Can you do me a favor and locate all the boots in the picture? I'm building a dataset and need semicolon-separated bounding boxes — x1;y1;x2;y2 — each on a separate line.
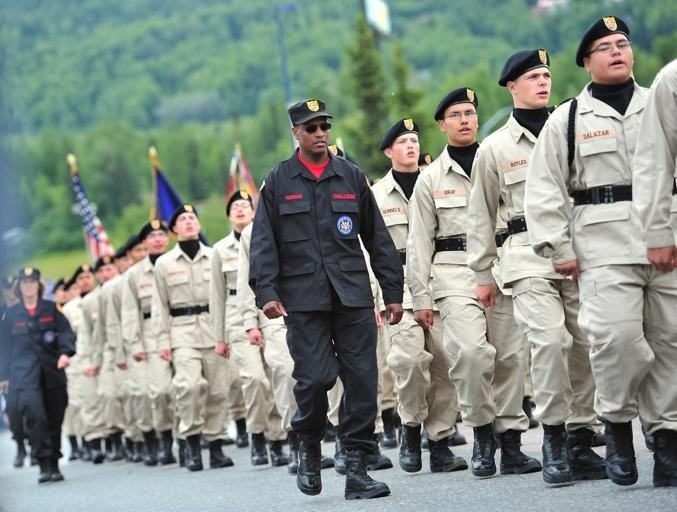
380;410;398;449
603;420;639;486
427;438;468;472
565;424;610;480
288;431;299;474
269;440;289;467
208;439;234;469
470;422;498;479
71;429;186;468
500;429;542;475
14;436;64;483
367;448;393;471
186;433;204;471
234;418;249;448
251;431;269;465
320;454;334;469
334;431;345;475
651;429;675;488
345;449;391;499
297;440;322;496
540;423;574;487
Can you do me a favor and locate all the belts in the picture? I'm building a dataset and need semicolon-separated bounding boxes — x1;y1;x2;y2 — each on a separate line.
170;305;208;317
143;312;151;319
229;289;236;295
395;185;633;266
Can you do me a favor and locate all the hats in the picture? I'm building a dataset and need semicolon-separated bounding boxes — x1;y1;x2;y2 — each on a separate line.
287;98;332;127
378;16;631;151
226;190;254;216
1;203;198;295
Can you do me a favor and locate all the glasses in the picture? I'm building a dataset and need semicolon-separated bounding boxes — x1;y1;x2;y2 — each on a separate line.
297;121;332;132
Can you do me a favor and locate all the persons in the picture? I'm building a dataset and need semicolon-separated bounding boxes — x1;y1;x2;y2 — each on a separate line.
50;220;187;467
468;48;607;480
407;87;543;478
208;191;289;467
629;58;677;271
521;15;677;489
2;267;76;480
150;204;234;471
360;117;469;475
249;99;406;500
237;221;335;475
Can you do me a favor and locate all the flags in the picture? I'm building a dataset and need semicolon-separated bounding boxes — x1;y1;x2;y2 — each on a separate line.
225;149;261;221
150;158;210;247
70;165;116;271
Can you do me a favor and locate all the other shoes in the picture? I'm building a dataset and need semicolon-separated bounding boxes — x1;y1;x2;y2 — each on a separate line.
324;424;336;442
591;430;605;447
447;424;468;446
523;408;540;429
